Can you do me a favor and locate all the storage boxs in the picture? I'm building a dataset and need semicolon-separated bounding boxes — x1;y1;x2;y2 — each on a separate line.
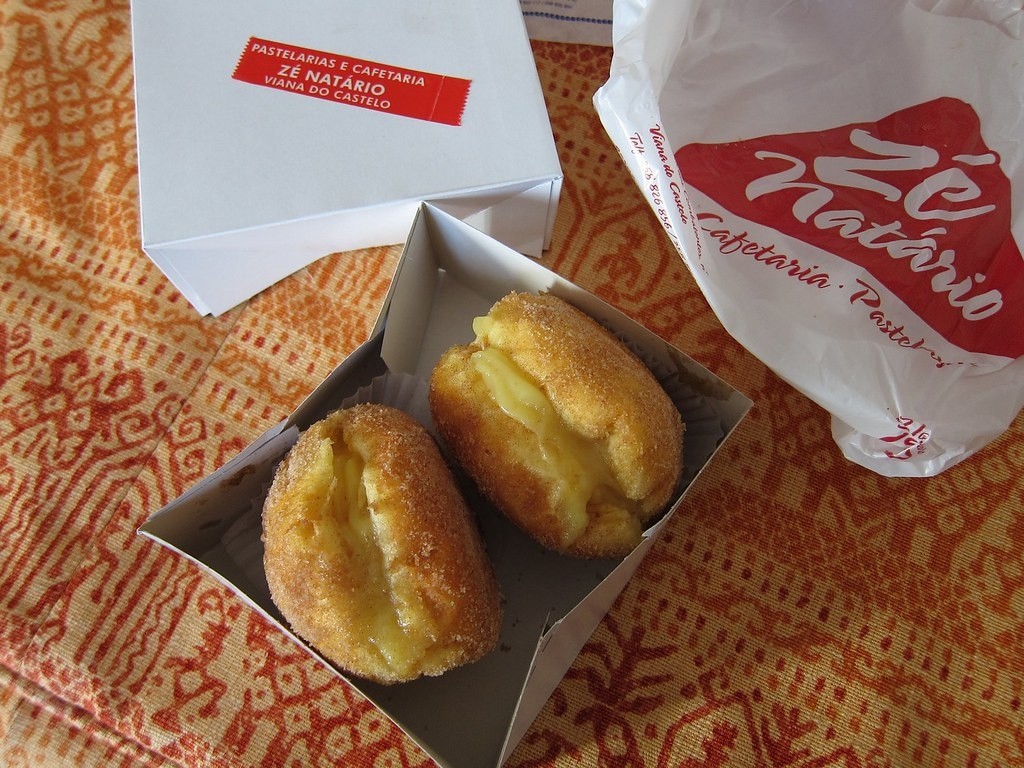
135;200;756;768
130;0;563;319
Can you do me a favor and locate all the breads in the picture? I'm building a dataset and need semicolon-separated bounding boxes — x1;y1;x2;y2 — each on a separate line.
431;294;686;562
259;402;502;685
